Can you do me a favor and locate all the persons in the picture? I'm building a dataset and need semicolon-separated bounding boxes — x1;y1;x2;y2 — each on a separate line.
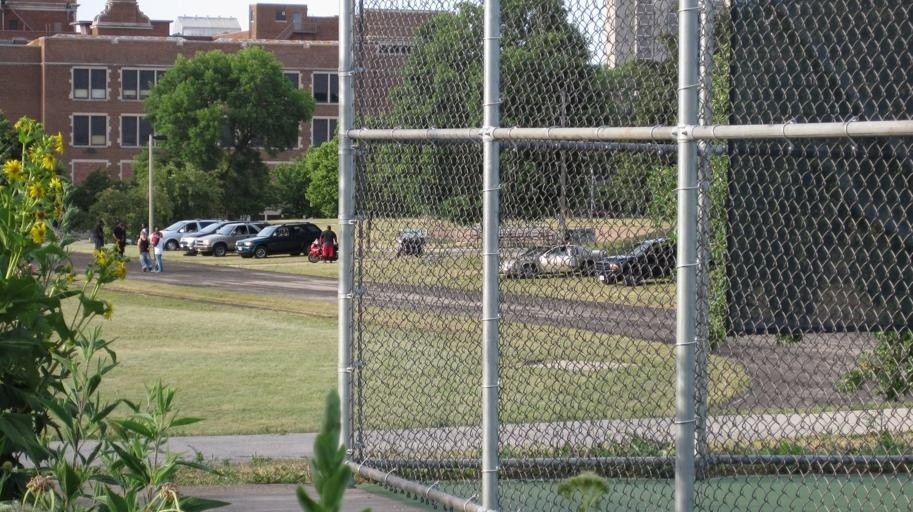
150;224;162;262
93;221;106;253
112;223;126;257
319;226;337;263
139;224;148;236
135;232;150;273
150;231;164;272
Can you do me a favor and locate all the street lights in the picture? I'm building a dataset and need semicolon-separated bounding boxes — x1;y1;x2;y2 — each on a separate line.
148;133;168;239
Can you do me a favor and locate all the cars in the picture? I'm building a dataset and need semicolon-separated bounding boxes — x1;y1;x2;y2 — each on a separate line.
499;239;679;285
163;219;323;260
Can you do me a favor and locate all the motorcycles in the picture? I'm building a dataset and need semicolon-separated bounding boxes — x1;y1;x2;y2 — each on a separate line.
308;239;338;263
